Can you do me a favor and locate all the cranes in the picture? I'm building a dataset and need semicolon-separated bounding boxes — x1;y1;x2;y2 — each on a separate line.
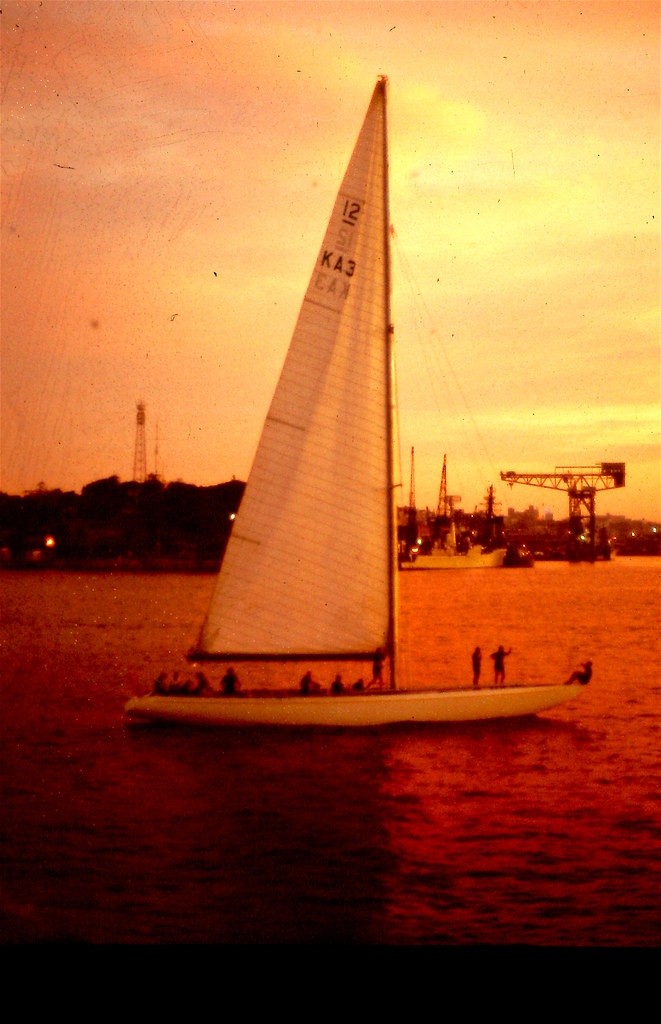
501;462;626;549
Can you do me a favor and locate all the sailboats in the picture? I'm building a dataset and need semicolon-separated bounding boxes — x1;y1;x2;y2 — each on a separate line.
125;72;585;727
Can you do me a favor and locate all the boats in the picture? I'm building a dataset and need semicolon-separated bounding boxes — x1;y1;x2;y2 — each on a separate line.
399;520;509;569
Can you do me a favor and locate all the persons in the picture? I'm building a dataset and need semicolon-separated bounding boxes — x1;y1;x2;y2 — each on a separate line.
564;661;593;686
351;681;363;690
489;644;512;687
471;645;483;686
300;671;312;692
220;667;237;696
331;675;345;695
366;646;386;689
153;669;208;697
